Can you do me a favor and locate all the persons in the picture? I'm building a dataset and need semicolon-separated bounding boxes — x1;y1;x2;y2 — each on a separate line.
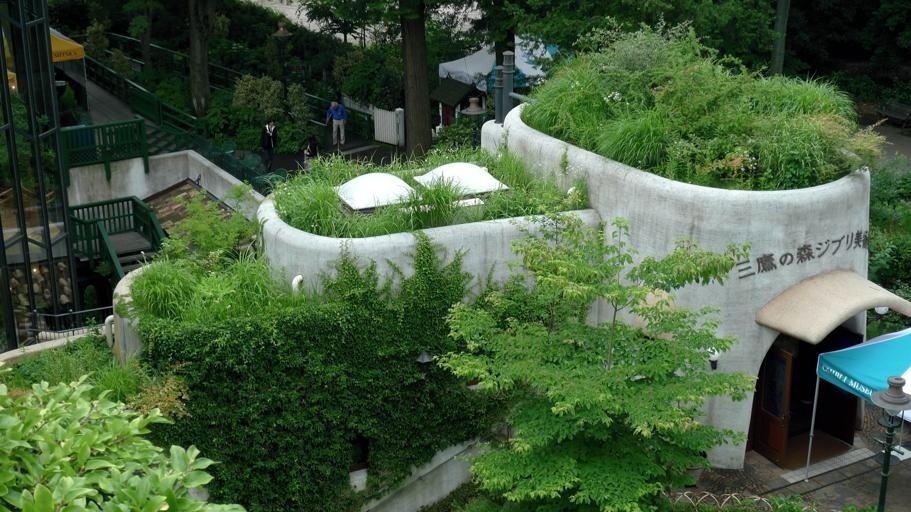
325;101;347;147
261;120;277;173
301;134;317;168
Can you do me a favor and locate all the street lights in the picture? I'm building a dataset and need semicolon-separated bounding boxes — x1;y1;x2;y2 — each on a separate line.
459;96;489;151
869;374;910;512
